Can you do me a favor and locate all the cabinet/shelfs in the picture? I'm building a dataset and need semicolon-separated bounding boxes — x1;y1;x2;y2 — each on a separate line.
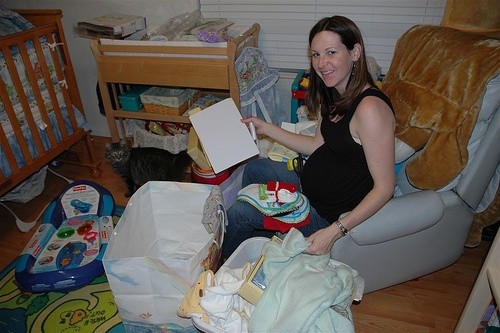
90;24;261;147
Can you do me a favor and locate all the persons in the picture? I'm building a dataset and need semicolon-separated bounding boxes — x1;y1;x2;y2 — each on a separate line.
213;14;397;275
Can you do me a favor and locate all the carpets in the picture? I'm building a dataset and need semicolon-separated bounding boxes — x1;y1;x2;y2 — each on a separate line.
0;204;126;333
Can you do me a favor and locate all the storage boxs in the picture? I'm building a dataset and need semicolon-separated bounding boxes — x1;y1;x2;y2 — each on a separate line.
118;86;150;112
140;85;203;115
73;14;136;40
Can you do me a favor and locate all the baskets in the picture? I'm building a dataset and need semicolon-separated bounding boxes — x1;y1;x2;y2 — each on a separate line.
140;88;203;115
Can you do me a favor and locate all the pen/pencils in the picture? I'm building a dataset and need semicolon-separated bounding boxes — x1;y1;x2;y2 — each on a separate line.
250;121;257;144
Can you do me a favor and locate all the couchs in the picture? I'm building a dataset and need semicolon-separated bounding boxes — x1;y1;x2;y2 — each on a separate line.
330;25;500;294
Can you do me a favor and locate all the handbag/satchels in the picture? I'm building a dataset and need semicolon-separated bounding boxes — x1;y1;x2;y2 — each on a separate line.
101;181;224;333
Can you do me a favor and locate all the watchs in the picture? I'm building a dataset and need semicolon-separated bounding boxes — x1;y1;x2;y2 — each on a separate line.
334;220;348;236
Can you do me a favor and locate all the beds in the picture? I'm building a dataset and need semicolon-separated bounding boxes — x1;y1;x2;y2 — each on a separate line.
0;8;100;198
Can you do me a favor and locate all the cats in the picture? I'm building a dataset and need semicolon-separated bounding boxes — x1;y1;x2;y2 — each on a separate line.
104;137;194;198
